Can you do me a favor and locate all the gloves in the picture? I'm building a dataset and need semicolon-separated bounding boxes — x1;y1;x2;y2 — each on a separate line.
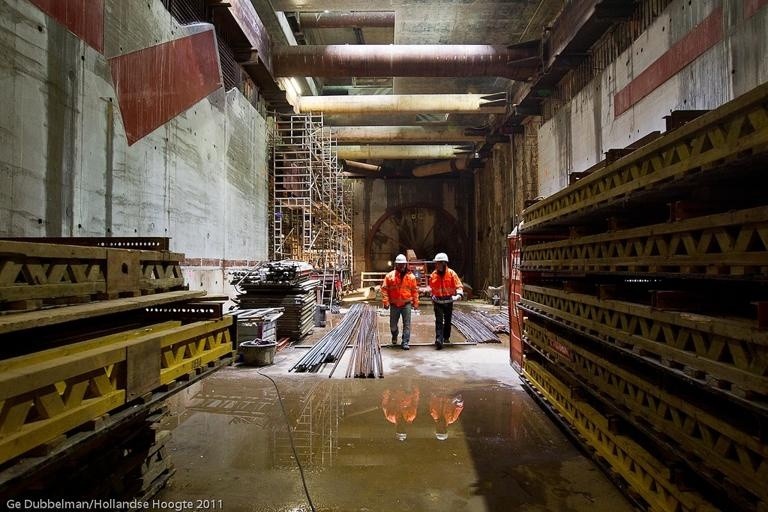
452;295;462;301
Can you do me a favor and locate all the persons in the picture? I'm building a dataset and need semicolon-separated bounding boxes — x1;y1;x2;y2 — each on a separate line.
428;252;463;349
380;254;419;350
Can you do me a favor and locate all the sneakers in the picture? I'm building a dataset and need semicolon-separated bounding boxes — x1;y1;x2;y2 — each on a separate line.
403;345;409;349
392;337;397;344
436;339;442;348
443;337;450;343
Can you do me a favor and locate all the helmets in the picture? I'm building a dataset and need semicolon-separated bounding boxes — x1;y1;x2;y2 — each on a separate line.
433;253;449;262
395;254;407;264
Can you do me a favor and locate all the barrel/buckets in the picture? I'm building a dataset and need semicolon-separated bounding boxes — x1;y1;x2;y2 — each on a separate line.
240;340;277;366
